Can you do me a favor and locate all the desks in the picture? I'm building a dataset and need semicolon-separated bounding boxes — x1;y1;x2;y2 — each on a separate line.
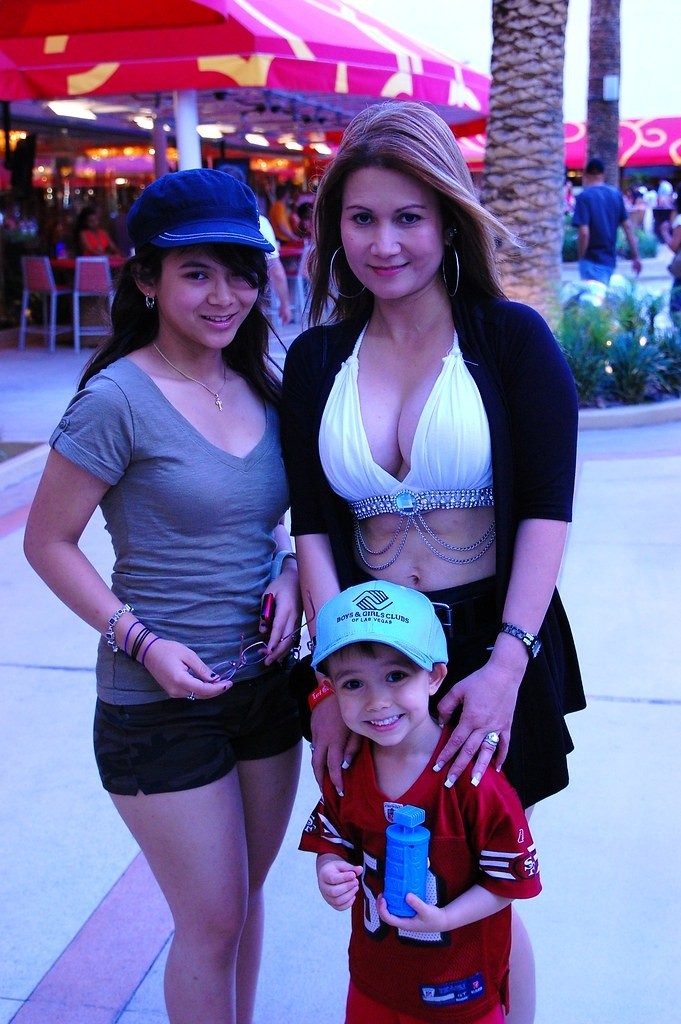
54;249;308;338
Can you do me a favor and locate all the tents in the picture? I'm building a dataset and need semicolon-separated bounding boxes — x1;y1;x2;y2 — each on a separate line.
0;0;681;172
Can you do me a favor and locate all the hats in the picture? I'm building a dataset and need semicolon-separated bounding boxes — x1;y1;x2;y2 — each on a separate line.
585;158;603;175
308;582;449;670
125;168;276;253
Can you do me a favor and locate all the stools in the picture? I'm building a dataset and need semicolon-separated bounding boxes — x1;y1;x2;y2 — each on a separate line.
18;258;71;352
72;258;117;352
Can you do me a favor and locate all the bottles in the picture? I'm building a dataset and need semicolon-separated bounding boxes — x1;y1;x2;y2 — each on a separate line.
56;243;66;257
383;805;430;917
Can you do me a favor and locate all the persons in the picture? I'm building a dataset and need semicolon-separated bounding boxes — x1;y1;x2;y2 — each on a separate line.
0;185;316;328
297;581;543;1024
281;100;586;1024
24;166;304;1024
564;157;681;332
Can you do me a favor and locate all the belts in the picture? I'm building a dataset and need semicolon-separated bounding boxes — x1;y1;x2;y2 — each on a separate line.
424;589;507;643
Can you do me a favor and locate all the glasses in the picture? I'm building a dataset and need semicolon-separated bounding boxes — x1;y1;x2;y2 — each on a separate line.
203;590;316;685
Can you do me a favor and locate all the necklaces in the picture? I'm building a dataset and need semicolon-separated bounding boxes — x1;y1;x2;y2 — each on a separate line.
153;341;228;412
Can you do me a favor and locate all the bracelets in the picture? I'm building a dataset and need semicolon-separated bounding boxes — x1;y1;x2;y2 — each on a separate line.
308;679;336;711
131;627;151;661
142;637;164;667
105;604;134;653
124;621;143;657
271;550;297;581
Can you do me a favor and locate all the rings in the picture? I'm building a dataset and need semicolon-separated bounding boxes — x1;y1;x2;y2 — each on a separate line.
187;691;195;701
484;731;500;746
309;743;315;751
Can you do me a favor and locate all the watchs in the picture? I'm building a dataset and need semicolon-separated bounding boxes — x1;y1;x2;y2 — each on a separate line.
499;622;541;659
